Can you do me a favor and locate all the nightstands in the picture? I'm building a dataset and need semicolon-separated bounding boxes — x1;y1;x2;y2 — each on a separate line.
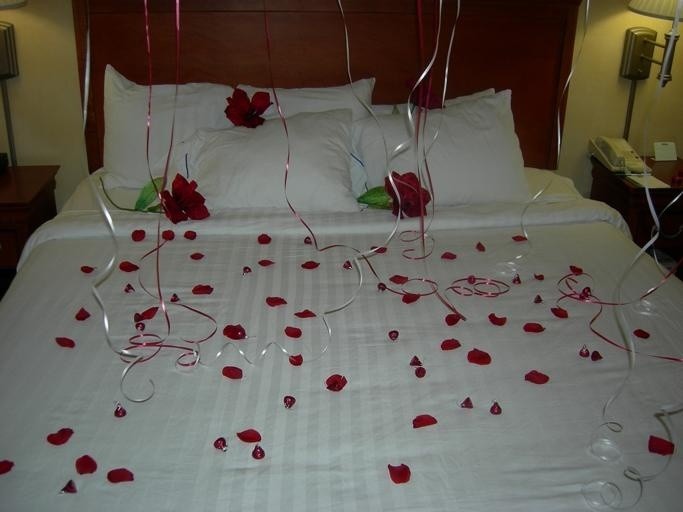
0;166;59;269
590;155;683;246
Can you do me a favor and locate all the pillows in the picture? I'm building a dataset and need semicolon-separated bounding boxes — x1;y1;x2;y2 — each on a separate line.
352;90;526;209
237;78;375;119
164;109;361;212
370;88;496;116
105;63;233;191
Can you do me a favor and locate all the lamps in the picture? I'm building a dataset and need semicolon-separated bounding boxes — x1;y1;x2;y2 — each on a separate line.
0;0;26;79
620;0;683;87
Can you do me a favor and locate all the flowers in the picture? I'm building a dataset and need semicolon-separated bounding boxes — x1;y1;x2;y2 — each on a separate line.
159;174;210;224
385;170;432;217
224;88;274;128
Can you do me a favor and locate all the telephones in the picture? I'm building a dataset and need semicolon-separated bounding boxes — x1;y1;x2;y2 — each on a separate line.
588;136;653;173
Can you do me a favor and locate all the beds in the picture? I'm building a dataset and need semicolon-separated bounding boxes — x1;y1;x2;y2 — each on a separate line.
0;0;683;512
